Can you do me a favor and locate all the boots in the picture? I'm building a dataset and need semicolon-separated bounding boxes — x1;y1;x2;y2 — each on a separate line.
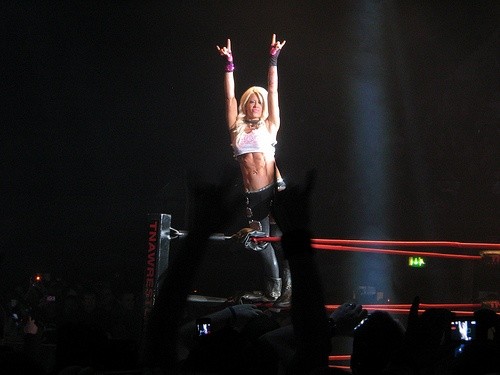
274;260;291;308
243;276;283;305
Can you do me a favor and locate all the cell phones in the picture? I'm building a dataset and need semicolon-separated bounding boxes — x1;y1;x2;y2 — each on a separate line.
196;317;212;338
352;314;370;335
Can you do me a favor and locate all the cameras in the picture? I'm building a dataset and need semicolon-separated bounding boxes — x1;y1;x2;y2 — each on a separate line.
448;316;480;343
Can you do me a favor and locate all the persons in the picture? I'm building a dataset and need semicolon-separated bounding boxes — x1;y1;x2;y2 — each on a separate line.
216;33;293;307
0;277;500;375
